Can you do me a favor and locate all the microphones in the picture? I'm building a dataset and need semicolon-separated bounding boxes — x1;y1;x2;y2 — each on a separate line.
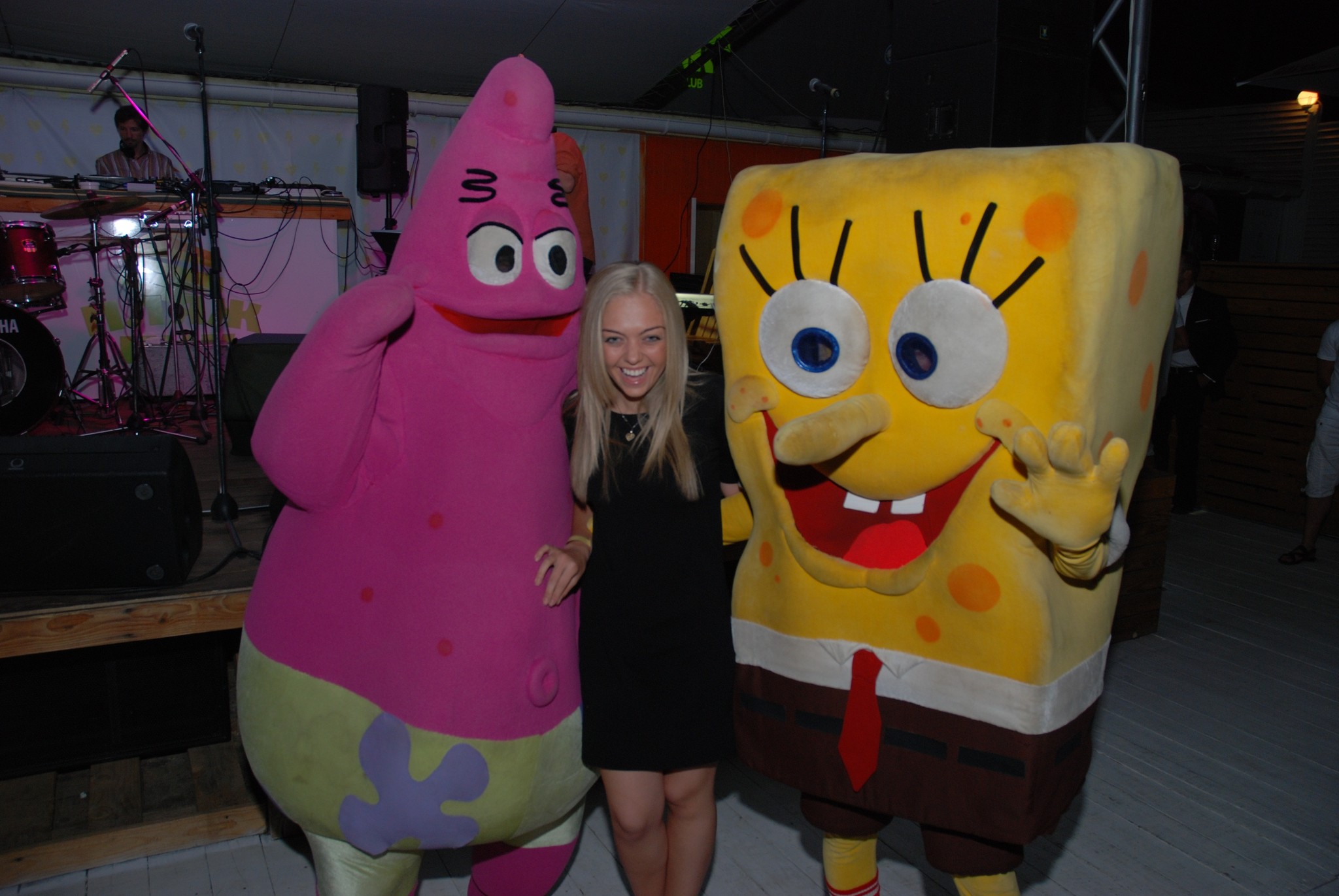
144;203;182;226
184;23;204;41
58;243;78;258
88;47;130;94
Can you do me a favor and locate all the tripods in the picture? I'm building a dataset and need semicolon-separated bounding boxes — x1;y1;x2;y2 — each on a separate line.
66;59;279;557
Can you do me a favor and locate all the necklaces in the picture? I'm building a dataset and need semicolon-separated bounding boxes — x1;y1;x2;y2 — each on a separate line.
616;402;649;441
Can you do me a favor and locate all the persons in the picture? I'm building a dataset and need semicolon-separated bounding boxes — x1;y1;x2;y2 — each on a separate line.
95;105;177;179
1276;320;1339;564
532;262;744;896
551;130;595;285
1158;254;1225;517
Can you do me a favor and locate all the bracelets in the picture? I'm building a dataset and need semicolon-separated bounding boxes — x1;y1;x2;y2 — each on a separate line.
568;535;592;551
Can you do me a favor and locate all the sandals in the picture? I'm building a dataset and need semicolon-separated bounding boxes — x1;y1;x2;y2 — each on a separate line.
1278;545;1317;565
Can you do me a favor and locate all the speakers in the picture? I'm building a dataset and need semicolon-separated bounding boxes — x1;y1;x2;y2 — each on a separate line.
222;333;308;457
0;434;205;592
355;82;411;194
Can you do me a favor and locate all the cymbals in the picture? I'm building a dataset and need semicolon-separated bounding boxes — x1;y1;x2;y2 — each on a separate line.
39;195;150;221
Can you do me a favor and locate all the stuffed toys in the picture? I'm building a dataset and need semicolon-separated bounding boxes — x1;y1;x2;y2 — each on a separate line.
720;143;1183;896
236;54;604;896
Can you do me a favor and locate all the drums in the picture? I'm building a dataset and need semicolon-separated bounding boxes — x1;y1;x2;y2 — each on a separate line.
0;297;69;440
0;218;69;305
